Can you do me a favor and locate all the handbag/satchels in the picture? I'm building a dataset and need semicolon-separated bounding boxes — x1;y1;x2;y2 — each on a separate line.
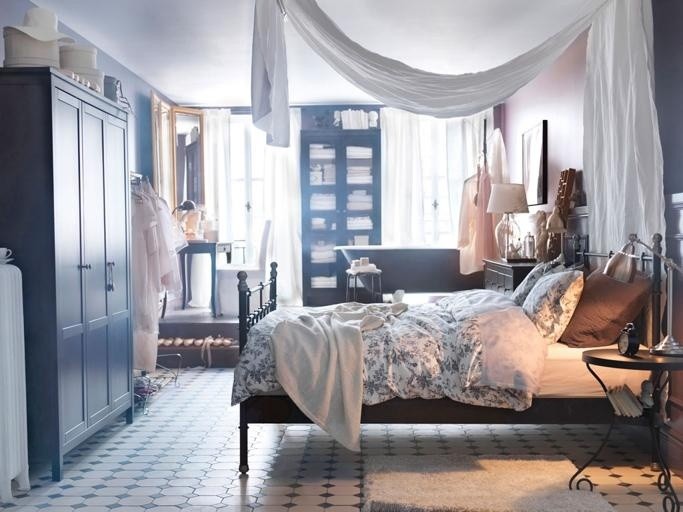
104;74;122;104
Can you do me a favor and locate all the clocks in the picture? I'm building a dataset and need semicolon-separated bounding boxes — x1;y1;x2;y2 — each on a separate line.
618;322;640;358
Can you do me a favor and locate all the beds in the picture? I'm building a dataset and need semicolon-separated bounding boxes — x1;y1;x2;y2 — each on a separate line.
237;233;663;474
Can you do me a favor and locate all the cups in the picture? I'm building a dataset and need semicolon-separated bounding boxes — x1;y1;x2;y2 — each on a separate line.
0;248;12;258
352;260;362;267
360;257;369;267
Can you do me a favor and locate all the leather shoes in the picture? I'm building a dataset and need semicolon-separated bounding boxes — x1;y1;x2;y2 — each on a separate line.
158;336;235;347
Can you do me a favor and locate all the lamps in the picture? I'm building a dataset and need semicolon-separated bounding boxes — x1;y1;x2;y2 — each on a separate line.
171;200;196;215
485;183;530;264
603;232;683;357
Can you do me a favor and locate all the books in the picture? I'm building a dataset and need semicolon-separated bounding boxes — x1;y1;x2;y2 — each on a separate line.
607;383;644;418
307;109;379;289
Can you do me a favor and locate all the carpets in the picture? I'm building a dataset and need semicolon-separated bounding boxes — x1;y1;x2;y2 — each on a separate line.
362;455;614;512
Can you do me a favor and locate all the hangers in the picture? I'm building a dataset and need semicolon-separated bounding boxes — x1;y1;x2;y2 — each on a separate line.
464;156;483;184
129;179;167;204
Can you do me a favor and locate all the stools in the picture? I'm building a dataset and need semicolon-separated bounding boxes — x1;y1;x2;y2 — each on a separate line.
346;272;381;309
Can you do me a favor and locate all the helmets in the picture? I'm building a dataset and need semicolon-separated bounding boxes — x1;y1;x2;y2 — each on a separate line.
7;7;77;43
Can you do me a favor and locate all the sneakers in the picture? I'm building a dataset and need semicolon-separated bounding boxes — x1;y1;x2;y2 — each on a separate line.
133;374;161;396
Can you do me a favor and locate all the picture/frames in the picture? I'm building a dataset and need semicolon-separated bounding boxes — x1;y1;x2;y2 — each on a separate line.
521;119;546;206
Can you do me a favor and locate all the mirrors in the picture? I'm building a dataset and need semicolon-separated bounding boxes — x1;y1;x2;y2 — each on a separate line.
149;91;206;221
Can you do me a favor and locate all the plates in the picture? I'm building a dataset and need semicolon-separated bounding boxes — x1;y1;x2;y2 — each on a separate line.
0;259;14;263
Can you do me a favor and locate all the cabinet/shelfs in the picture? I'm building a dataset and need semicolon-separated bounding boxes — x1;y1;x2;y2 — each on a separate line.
301;128;381;310
483;259;537;297
0;66;135;482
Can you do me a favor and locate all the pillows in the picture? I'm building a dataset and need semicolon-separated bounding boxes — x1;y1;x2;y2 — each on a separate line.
512;253;657;348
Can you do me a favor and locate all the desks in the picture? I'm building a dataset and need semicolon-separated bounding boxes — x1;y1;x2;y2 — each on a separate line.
569;349;683;512
176;243;231;316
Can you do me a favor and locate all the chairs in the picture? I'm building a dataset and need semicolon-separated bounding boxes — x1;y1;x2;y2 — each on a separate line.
216;220;271;316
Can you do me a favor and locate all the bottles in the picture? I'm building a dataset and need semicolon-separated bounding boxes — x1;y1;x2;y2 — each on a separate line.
524;232;534;259
